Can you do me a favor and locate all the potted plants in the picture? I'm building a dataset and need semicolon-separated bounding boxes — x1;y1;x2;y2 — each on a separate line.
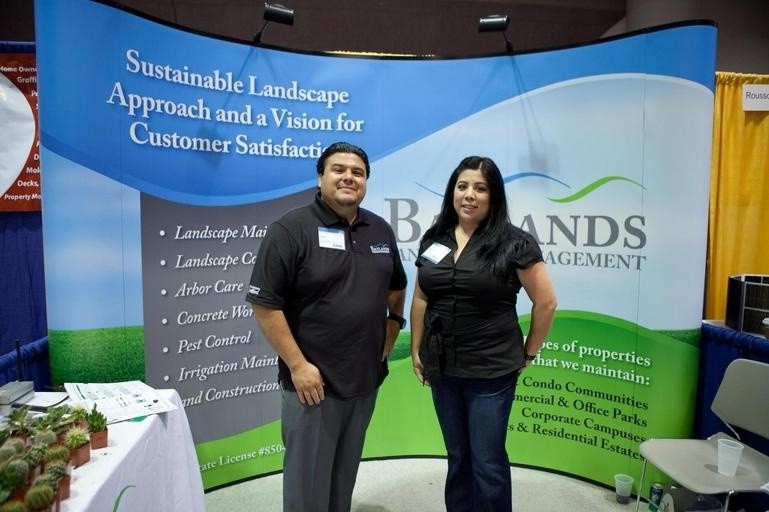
1;400;109;511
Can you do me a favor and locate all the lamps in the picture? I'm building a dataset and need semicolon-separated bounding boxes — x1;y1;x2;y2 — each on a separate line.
253;2;295;45
477;14;515;53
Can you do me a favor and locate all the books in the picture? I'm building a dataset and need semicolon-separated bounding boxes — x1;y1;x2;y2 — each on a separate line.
11;389;70;413
64;379;178;428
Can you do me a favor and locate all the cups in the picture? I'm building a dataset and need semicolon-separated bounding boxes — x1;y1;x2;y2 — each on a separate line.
614;474;634;506
716;438;744;477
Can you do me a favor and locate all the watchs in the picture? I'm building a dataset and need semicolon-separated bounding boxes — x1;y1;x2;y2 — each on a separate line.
388;312;407;331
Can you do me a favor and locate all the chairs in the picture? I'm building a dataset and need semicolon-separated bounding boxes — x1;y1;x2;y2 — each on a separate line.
636;359;769;512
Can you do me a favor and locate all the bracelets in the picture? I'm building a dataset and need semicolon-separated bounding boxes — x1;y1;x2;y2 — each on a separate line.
524;351;536;363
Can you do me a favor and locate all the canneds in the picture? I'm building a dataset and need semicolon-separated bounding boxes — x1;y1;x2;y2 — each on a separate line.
649;483;664;512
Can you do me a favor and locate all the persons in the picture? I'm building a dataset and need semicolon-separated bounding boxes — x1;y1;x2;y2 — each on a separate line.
247;140;408;511
408;155;559;512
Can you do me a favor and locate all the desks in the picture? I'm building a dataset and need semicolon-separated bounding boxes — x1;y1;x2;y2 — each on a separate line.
695;318;769;458
2;388;204;512
